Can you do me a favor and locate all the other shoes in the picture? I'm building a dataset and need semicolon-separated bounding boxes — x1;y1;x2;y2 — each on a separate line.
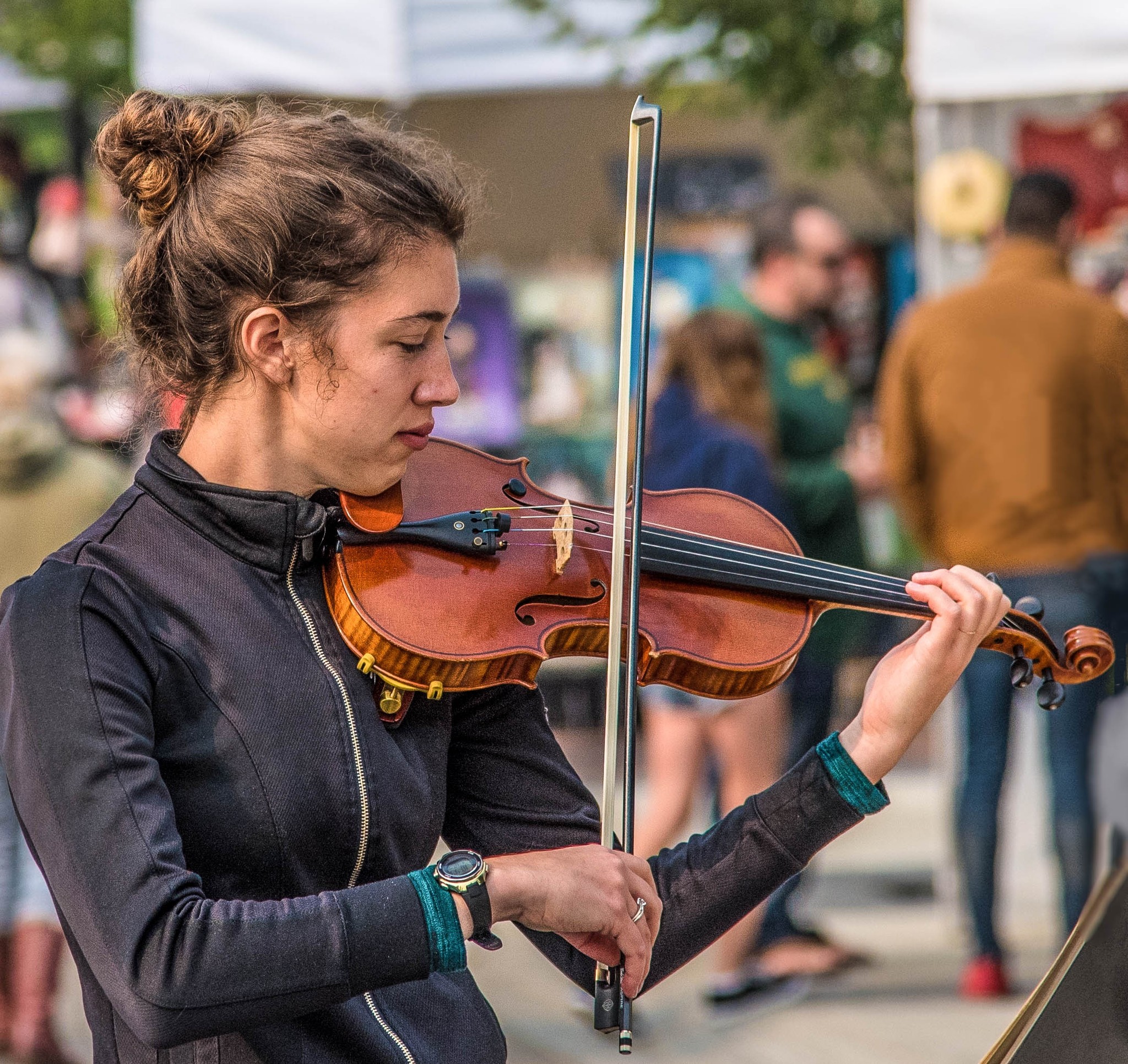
568;992;641;1042
962;960;1008;999
701;975;814;1029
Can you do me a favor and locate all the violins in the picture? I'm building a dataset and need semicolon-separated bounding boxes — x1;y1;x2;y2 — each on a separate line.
314;434;1114;715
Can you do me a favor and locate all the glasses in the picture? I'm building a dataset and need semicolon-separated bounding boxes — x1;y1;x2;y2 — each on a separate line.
792;244;844;273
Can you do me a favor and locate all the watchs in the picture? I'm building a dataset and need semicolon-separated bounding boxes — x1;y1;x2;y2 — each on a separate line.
434;847;504;951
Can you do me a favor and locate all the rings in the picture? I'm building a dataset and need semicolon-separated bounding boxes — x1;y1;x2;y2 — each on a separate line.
632;897;647;922
958;628;977;635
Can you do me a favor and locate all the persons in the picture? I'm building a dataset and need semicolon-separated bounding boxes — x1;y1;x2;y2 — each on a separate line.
0;126;1128;1064
1;85;1011;1064
